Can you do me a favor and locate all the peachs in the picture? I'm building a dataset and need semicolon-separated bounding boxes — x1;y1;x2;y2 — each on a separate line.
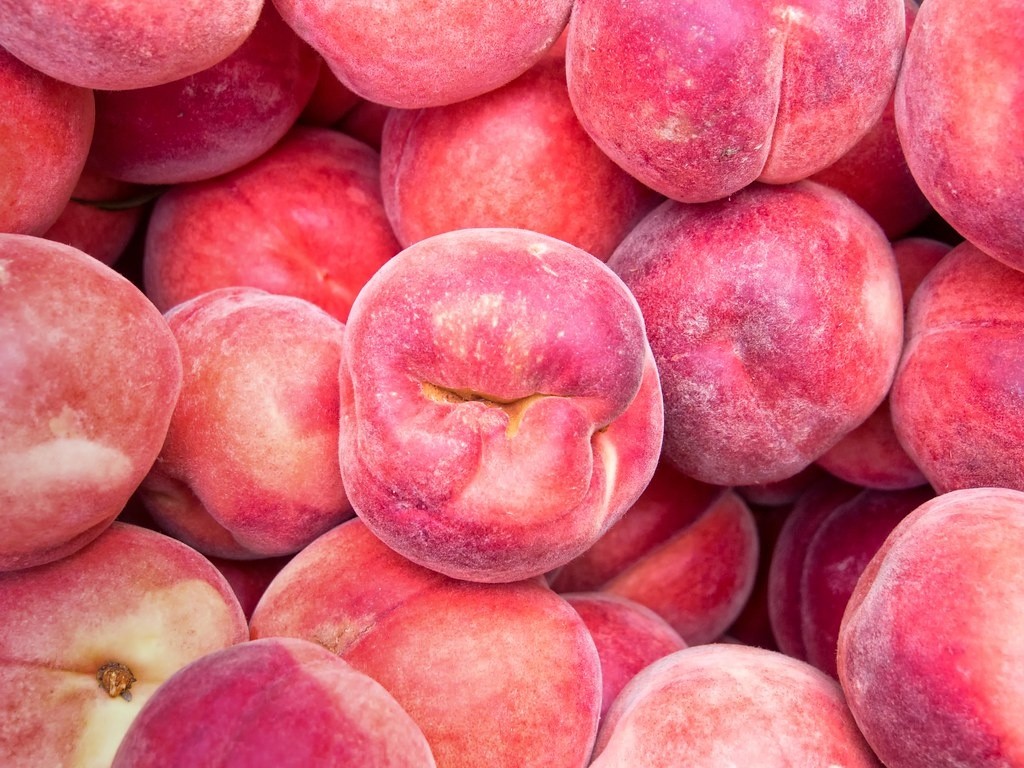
0;0;1024;768
